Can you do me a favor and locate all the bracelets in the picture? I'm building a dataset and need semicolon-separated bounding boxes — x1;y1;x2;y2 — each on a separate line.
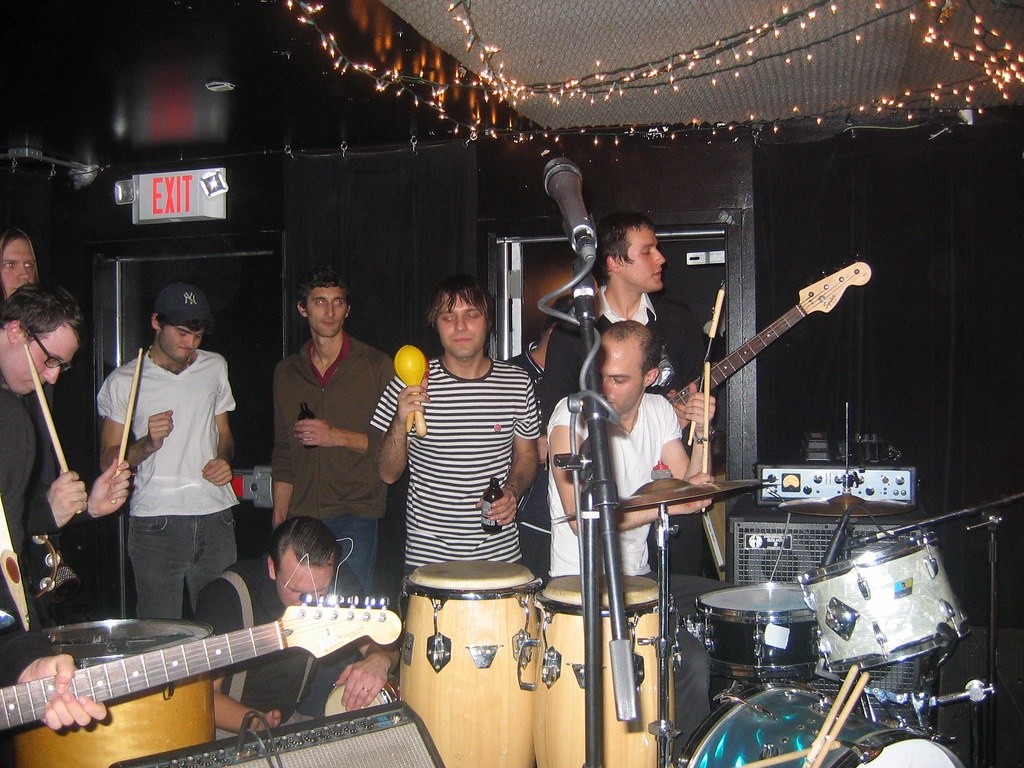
693;423;715;444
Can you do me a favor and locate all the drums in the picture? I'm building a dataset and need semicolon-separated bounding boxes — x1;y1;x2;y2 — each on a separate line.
532;573;679;768
695;579;827;679
798;530;971;674
678;681;967;768
324;676;400;717
400;559;539;768
11;616;218;767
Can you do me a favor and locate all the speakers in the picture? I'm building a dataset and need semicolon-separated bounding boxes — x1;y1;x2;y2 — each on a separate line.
725;492;930;729
109;700;446;768
938;627;1024;768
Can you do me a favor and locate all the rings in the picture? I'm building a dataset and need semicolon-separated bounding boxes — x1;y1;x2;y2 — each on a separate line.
363;687;370;692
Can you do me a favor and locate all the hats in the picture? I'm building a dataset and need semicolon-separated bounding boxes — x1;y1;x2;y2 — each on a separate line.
154;282;215;325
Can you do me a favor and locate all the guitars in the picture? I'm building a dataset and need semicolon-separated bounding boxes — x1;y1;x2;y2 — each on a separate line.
668;254;872;410
0;592;403;734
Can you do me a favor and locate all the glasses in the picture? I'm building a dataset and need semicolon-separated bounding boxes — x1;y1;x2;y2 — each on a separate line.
29;324;72;374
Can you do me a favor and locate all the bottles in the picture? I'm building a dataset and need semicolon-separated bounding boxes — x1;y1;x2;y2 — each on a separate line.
297;402;319;449
481;478;505;535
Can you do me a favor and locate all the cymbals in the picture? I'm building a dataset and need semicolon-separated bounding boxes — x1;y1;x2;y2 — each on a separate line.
780;494;919;518
549;474;777;524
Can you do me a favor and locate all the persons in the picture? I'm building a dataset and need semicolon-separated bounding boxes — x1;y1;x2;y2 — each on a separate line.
548;320;740;768
544;211;705;578
270;264;395;609
0;221;130;627
504;295;574;590
0;556;106;768
369;273;539;624
97;281;240;620
195;516;399;742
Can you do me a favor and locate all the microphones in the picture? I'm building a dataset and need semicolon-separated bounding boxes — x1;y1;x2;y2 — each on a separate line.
537;143;597;262
820;506;853;568
